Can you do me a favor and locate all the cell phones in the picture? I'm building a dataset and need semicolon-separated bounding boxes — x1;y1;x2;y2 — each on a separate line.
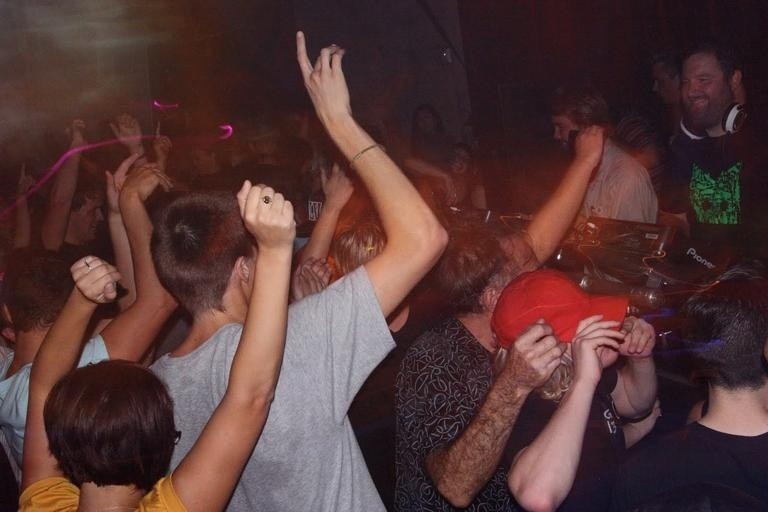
568;131;578;152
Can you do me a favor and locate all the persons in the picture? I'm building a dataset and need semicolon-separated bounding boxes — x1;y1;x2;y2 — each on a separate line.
149;30;449;510
103;116;149;164
404;103;489;214
601;104;662;174
614;270;768;510
289;256;337;301
17;180;296;512
549;86;657;229
293;160;356;261
151;120;174;168
327;222;439;512
32;117;110;287
651;39;768;267
491;270;657;510
394;219;568;512
3;160;38;253
2;162;180;485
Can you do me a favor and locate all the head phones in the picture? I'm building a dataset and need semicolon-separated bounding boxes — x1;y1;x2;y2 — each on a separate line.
679;103;750;141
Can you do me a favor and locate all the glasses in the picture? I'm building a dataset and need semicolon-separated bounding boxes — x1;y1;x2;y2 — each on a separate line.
173;431;182;446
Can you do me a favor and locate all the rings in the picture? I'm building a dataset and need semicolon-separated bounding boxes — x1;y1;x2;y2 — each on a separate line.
258;195;274;205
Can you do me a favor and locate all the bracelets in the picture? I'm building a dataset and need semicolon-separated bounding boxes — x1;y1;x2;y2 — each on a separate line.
344;142;394;158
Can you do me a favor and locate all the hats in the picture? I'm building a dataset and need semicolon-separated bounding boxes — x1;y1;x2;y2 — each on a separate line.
491;271;628;348
